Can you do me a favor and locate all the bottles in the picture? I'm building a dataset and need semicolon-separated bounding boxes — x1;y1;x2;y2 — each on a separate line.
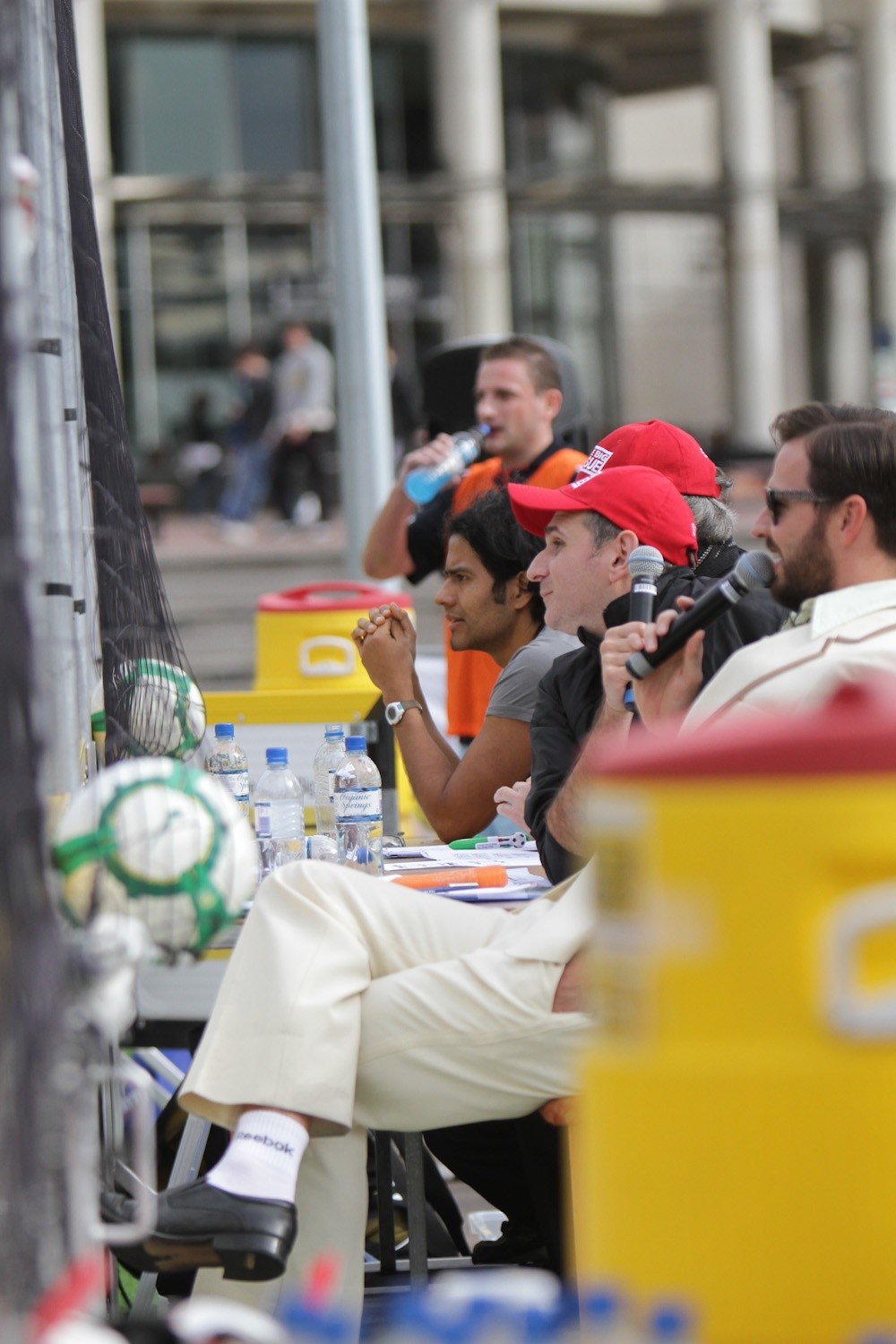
205;724;249;819
332;735;383;875
402;423;490;505
313;725;347;840
253;748;304;867
256;834;371;875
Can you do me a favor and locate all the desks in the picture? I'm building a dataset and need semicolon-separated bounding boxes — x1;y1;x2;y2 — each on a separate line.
109;835;551;1289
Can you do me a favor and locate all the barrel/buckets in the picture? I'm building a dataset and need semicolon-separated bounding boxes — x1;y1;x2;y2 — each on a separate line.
577;714;896;1343
252;582;417;820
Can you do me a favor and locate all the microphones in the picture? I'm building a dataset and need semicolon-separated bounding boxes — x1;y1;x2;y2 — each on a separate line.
624;545;666;712
627;548;776;683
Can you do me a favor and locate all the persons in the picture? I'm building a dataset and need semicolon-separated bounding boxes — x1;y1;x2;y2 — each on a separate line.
493;422;747;843
210;313;428;523
496;462;778;893
352;485;586;842
364;333;597;760
90;405;895;1344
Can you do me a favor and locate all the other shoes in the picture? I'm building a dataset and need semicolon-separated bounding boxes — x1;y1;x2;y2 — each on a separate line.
471;1218;560;1271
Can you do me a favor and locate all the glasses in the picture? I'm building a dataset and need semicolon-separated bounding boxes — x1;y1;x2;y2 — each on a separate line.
763;486;863;526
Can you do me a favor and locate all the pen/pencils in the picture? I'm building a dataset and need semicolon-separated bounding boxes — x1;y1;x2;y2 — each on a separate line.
449;831;530;850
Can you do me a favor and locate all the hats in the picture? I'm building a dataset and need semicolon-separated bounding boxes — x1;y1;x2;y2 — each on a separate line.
573;420;722;501
507;466;699;567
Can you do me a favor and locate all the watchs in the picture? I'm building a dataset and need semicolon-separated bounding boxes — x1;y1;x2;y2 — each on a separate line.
385;697;422;725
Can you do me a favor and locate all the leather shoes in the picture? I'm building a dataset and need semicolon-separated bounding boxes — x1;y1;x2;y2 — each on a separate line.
101;1178;298;1283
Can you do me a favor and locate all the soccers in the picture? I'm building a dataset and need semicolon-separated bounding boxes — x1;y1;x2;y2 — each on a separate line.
90;658;206;764
48;756;258;968
